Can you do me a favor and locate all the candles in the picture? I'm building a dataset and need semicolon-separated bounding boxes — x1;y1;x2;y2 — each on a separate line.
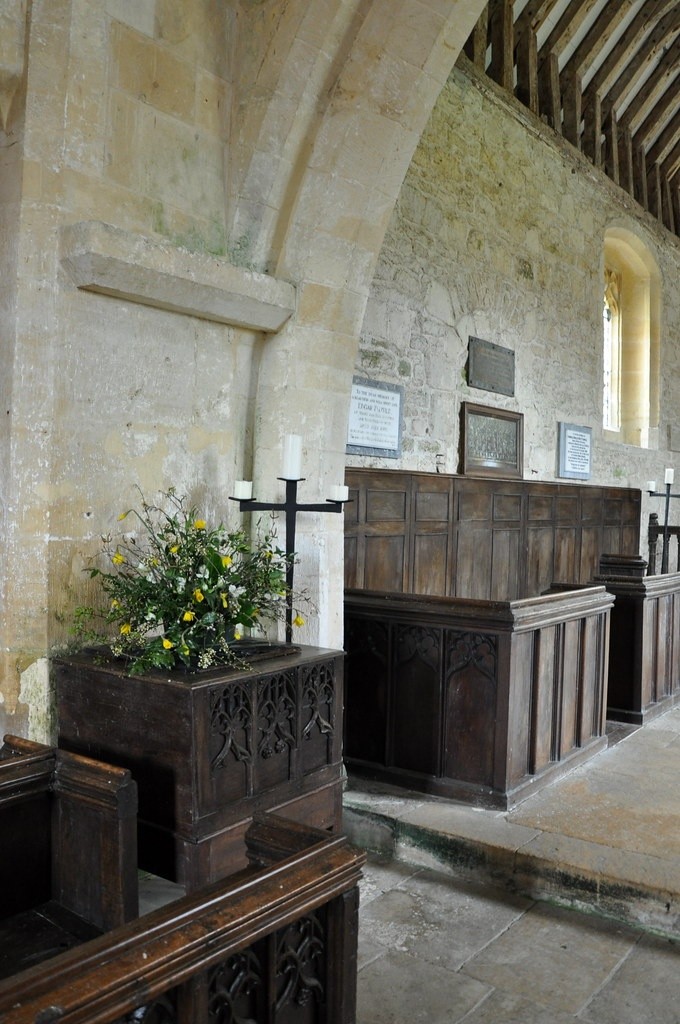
647;481;655;491
233;478;253;499
665;469;674;484
328;484;349;501
282;431;304;480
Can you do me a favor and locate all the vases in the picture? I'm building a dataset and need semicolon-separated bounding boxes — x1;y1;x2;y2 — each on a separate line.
50;633;350;885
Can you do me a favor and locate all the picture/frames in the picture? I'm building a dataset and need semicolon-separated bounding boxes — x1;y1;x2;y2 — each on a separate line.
461;401;524;481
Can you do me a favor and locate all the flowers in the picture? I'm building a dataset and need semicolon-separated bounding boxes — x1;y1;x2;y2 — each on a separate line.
55;481;322;680
